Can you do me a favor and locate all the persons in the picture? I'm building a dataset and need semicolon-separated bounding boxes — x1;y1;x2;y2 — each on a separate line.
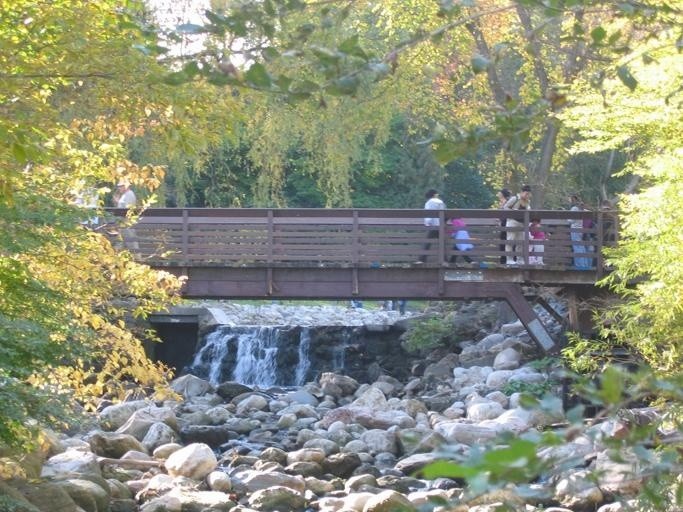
112;179;141;261
504;186;530;264
418;189;449;263
592;200;615;270
529;219;545;265
346;236;408;315
579;202;594;270
74;176;99;232
497;189;517;264
449;219;476;264
559;196;588;270
